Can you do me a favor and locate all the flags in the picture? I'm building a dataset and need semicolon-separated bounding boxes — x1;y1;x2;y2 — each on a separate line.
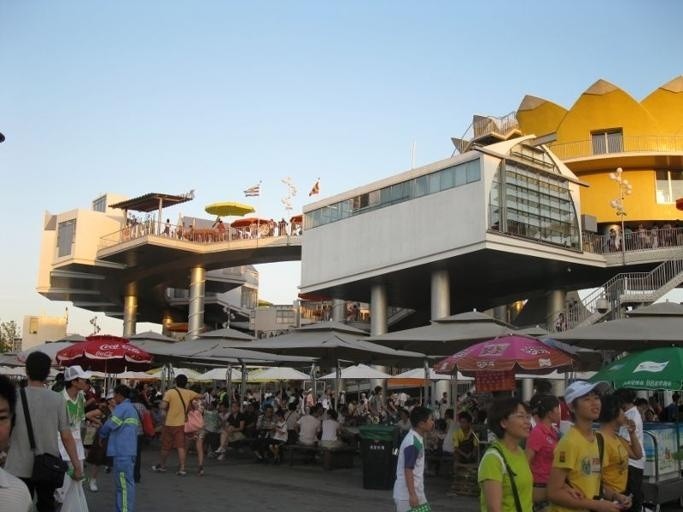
309;181;321;197
243;184;260;198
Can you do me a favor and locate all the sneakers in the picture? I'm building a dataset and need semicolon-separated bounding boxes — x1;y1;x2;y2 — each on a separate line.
206;446;226;461
176;464;204;476
152;464;167;472
256;455;281;465
88;466;112;492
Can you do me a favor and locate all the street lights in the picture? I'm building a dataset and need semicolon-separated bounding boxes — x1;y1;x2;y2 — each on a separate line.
608;167;632;266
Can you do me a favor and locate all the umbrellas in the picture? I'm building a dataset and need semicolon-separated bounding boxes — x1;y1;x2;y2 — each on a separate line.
205;201;258;241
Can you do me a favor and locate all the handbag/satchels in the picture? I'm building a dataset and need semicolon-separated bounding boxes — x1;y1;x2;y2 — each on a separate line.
184;410;204;433
144;411;155;436
60;478;89;512
32;453;69;488
83;426;97;445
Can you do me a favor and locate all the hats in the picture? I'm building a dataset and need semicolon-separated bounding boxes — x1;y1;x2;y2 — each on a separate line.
564;381;611;405
64;365;91;382
106;389;114;400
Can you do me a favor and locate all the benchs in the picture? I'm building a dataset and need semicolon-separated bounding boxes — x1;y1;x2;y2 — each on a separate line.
426;455;450;476
226;440;253;460
285;444;355;469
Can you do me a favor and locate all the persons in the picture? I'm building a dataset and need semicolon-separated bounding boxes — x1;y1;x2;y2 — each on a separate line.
451;379;682;511
393;406;435;511
1;345;207;512
582;217;683;255
203;385;464;465
126;211;302;244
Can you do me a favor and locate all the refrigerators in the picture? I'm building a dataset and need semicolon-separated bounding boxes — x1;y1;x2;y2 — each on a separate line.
590;420;682;476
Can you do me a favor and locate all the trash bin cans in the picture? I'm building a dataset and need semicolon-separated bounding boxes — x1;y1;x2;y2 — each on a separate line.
359;426;399;491
